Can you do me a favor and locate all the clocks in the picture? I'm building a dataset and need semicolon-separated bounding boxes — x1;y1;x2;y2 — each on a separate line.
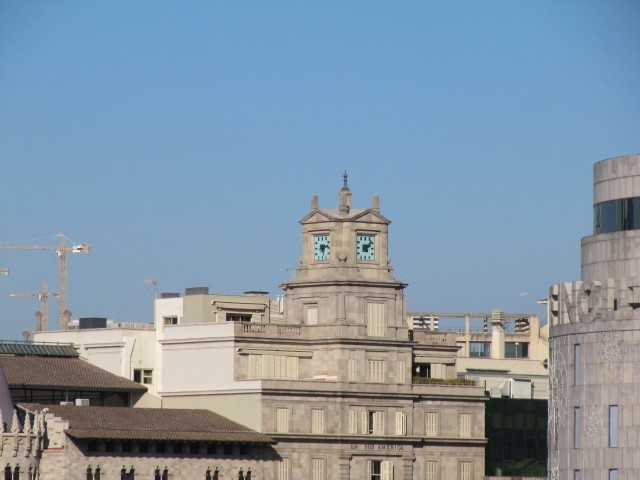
356;235;375;260
314;235;331;260
